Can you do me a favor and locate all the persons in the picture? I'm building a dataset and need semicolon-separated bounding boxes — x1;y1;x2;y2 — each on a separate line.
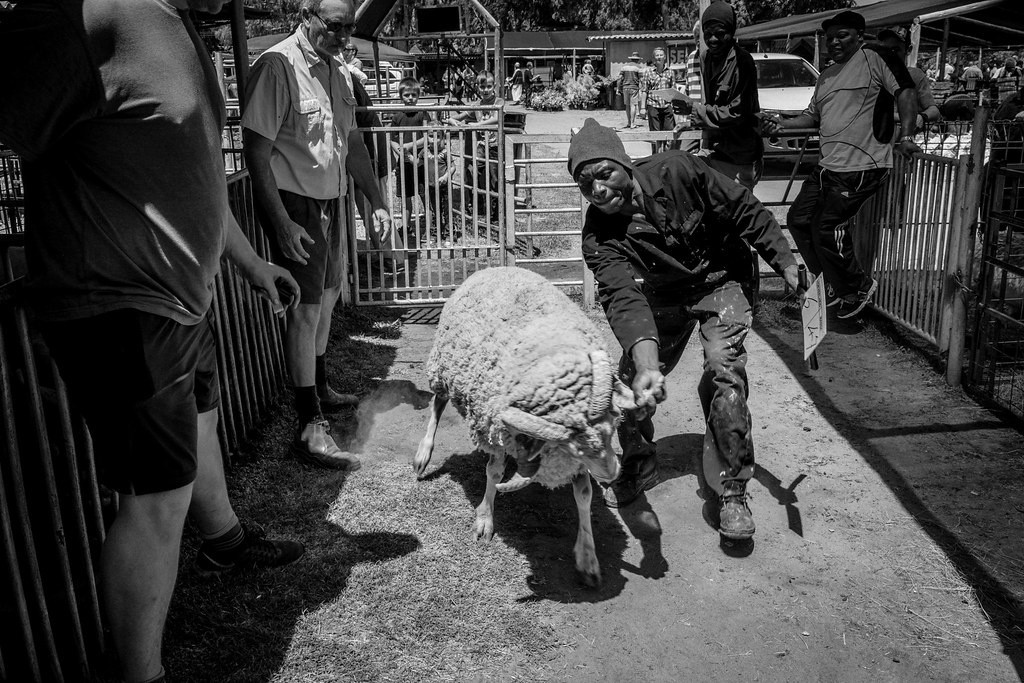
927;51;1024;98
764;10;921;323
388;70;507;238
640;47;676;154
582;59;595;77
443;62;541;106
238;0;417;470
0;0;300;683
567;117;816;539
672;0;763;313
617;51;657;127
850;30;940;273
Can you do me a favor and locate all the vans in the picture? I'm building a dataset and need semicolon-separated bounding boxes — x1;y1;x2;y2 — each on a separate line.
748;53;822;167
361;61;406;98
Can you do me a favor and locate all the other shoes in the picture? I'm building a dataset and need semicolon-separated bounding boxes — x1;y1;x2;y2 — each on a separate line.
419;234;427;243
397;224;413;239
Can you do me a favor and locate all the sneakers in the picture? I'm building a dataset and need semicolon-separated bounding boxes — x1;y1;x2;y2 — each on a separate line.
290;420;361;470
719;495;756;541
604;468;661;506
314;382;359;418
824;270;855;307
837;276;878;318
194;523;306;578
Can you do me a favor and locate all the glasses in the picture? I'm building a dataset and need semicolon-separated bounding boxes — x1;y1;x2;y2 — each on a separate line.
306;10;357;34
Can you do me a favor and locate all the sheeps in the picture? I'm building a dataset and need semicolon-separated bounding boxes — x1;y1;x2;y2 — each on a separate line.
410;266;652;590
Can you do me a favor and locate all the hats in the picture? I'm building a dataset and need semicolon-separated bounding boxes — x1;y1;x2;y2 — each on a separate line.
567;118;632;185
702;1;737;35
877;25;912;47
822;10;866;32
627;51;644;60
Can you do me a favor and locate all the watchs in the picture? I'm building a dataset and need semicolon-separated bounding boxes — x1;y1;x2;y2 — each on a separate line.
920;113;927;122
901;135;915;143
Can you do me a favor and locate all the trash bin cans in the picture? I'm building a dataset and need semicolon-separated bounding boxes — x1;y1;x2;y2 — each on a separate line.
462;112;527;215
610;80;626;110
